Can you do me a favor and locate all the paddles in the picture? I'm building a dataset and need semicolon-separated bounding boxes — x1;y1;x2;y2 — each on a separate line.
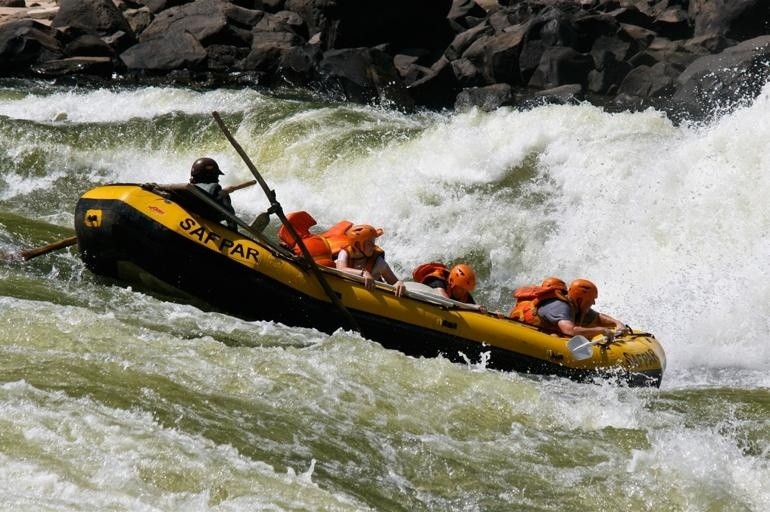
566;324;633;360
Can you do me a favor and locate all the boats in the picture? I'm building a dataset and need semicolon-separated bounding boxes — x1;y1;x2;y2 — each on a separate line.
71;177;669;390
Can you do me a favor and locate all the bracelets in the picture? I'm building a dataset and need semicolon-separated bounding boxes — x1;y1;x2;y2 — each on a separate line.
361;271;368;277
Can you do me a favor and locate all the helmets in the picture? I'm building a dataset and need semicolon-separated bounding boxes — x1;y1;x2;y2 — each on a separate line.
569;279;597;303
542;278;566;289
191;158;225;176
349;225;376;256
448;265;476;290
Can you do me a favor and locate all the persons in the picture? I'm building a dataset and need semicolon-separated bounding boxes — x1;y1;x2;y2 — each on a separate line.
411;261;476;301
332;224;407;298
533;277;631;342
508;276;568;324
189;157;239;233
